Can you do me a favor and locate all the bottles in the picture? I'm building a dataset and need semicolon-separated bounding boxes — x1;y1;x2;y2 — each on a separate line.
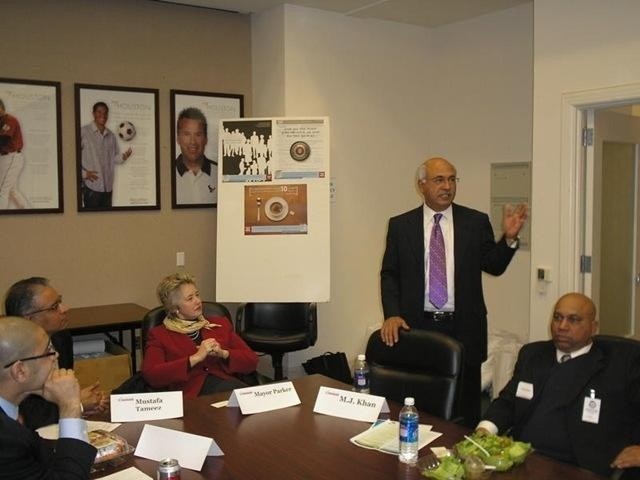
398;396;418;466
354;354;369;395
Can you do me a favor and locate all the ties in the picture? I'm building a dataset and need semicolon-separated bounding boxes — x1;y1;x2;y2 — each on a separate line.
561;355;570;363
429;213;448;310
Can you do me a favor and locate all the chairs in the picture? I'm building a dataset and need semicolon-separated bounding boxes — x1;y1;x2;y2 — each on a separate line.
236;290;319;382
363;327;467;422
587;333;639;365
141;299;234;378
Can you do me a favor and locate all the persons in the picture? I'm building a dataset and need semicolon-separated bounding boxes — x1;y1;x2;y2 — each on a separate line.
475;292;640;470
0;100;31;210
142;271;259;400
80;101;132;208
174;107;218;204
5;277;110;433
381;157;529;429
0;315;97;480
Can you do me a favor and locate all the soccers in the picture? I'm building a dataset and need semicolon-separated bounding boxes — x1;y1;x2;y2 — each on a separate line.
118;121;137;142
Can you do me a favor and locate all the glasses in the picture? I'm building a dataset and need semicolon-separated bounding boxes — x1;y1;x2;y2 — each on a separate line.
431;177;461;183
4;344;56;369
26;294;63;316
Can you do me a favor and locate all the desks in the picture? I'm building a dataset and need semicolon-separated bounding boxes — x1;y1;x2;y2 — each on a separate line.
32;373;602;480
46;299;152;366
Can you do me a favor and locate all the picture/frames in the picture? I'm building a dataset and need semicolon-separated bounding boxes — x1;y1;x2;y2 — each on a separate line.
74;82;161;212
170;89;245;210
0;75;65;215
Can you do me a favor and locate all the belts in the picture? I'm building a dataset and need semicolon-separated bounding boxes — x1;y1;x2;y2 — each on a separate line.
423;313;457;323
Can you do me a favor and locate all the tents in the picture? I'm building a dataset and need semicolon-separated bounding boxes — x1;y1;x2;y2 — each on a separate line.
186;330;201;341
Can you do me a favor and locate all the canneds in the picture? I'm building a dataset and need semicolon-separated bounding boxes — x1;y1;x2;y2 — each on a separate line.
157;458;180;480
